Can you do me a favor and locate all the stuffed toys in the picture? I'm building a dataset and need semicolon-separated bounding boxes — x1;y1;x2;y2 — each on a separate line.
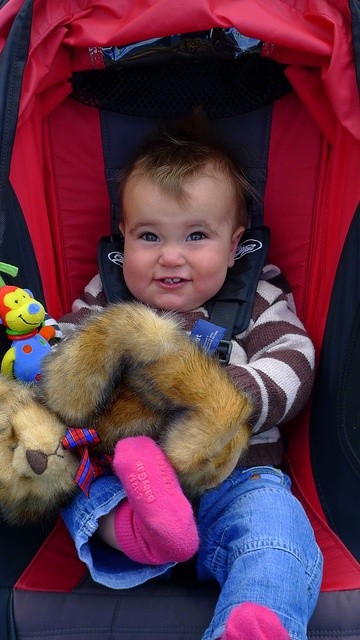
0;300;239;528
0;255;67;389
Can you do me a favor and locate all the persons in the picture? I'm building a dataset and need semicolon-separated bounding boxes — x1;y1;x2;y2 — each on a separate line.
48;129;324;640
